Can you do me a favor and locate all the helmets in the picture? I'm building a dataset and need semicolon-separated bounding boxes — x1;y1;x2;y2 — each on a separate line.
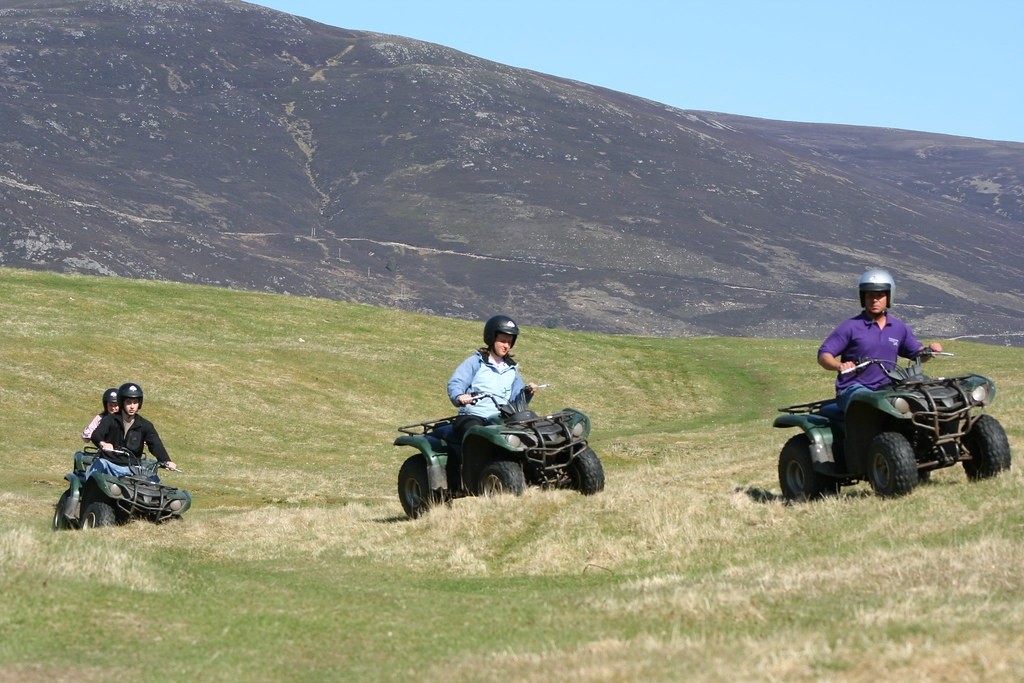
103;389;118;415
116;383;143;417
859;269;895;317
484;314;518;358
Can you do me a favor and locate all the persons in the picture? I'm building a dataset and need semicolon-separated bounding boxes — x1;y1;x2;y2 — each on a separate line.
447;315;539;449
85;383;176;482
82;387;121;444
818;268;943;420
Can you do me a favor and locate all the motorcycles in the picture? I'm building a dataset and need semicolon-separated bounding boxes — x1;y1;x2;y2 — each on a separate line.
52;436;192;533
773;346;1010;505
393;381;606;521
